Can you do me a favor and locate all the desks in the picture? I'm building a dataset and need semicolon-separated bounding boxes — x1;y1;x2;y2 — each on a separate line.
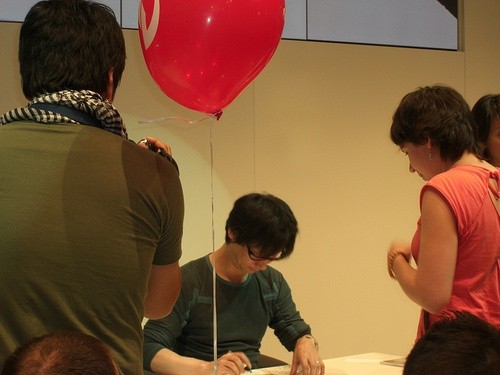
246;353;407;375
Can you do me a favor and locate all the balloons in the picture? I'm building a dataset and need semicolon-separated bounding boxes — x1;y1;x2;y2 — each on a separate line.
138;0;286;120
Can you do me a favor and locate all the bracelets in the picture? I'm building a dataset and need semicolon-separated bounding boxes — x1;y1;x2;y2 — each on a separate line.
296;335;319;351
388;250;409;278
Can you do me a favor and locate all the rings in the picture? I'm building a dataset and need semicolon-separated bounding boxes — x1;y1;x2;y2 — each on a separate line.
315;360;320;363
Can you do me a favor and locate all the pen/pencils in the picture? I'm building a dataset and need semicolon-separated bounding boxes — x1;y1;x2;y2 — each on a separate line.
226;351;252;375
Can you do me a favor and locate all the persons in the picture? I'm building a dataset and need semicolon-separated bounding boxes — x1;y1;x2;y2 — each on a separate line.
4;329;119;375
0;0;185;375
142;192;325;375
471;94;500;167
387;85;500;341
402;312;500;375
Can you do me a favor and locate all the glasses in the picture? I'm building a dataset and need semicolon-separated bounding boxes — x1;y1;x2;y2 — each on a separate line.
247;244;284;261
